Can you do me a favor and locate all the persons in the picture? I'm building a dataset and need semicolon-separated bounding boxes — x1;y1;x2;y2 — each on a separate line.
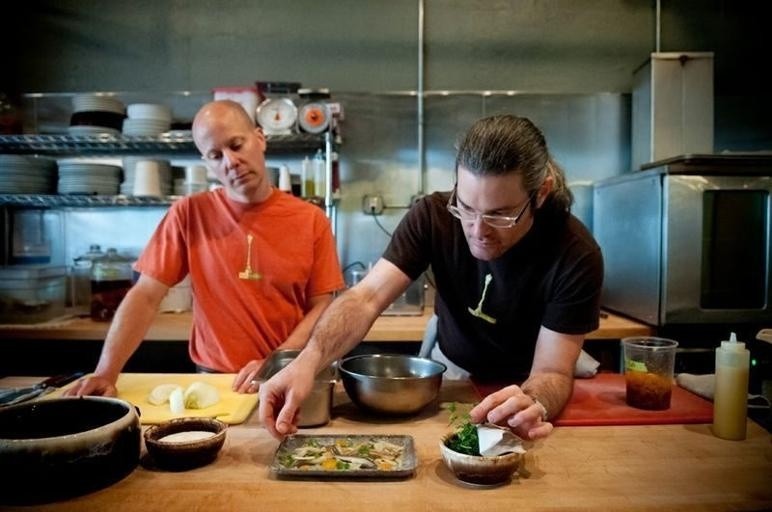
259;115;604;445
61;100;345;398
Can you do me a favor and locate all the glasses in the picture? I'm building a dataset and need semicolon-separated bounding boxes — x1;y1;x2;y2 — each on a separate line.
447;183;536;228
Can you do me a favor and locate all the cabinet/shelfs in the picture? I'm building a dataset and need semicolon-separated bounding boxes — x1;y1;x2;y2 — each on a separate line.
1;88;339;301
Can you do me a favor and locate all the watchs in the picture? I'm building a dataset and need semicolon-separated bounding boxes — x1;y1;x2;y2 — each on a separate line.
532;398;549;422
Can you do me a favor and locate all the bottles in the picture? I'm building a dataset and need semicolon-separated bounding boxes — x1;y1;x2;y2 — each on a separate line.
72;245;103;319
712;332;750;442
92;250;133;321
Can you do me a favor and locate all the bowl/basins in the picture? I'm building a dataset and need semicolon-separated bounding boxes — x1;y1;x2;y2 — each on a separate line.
132;161;171;198
250;347;337;429
337;353;449;418
121;103;173;140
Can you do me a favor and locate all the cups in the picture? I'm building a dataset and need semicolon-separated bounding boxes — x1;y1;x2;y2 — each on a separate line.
620;336;679;411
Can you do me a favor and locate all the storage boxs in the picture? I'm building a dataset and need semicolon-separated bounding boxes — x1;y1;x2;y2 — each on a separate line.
0;275;68;324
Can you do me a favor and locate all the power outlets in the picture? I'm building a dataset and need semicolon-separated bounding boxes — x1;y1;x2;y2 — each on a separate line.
364;193;384;214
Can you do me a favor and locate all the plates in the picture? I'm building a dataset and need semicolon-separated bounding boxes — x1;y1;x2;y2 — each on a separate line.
67;93;127;136
0;153;58;194
121;156;170;197
252;95;299;131
298;102;333;134
56;163;120;195
267;432;420;482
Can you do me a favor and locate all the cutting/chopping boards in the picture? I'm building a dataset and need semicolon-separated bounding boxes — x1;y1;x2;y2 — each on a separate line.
32;372;260;426
467;370;714;426
